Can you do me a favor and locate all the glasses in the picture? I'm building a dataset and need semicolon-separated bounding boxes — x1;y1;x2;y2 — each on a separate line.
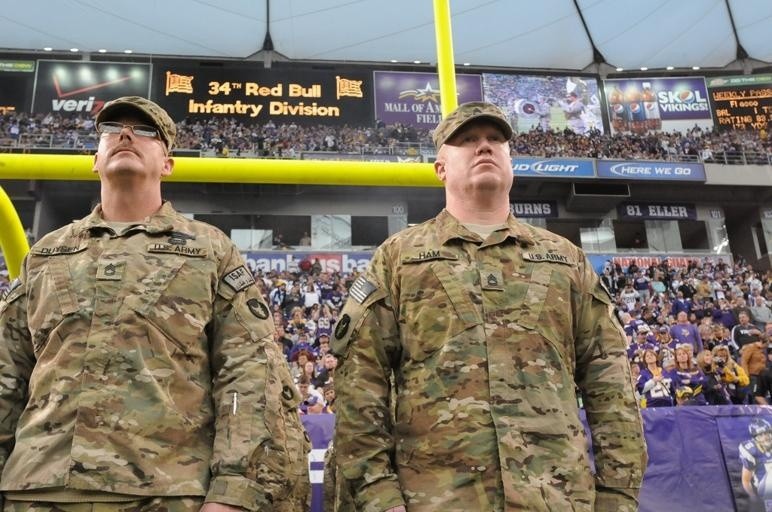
98;121;162;142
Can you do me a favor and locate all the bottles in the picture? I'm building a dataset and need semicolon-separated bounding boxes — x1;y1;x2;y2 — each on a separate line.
609;80;663;135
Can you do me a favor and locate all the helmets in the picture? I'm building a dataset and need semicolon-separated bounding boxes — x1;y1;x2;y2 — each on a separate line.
748;417;772;459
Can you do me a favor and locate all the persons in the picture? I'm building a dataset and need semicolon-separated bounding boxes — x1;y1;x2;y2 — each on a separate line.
483;74;602;134
173;115;435;160
509;121;771;164
598;255;771;409
2;110;98;155
244;231;370;415
738;418;772;512
0;94;313;512
322;100;651;512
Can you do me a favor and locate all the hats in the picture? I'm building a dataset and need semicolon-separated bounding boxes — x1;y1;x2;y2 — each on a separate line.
94;95;178;157
430;101;514;154
